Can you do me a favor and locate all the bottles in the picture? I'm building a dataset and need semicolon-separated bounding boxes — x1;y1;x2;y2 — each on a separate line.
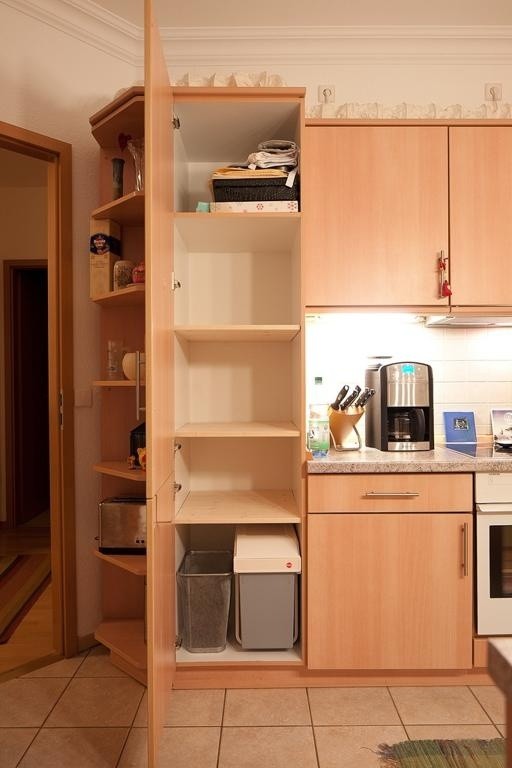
308;377;331;458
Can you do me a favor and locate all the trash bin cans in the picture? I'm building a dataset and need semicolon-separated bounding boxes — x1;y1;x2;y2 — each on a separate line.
178;549;233;655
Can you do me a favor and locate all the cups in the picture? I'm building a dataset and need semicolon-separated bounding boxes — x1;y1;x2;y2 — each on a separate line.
107;340;129;380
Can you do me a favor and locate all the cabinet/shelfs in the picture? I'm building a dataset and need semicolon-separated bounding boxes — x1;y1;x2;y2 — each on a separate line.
304;119;448;319
146;0;310;766
90;84;146;688
308;468;476;685
449;120;511;314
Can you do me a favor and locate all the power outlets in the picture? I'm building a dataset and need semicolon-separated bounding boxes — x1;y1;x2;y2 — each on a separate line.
318;85;335;104
485;83;502;101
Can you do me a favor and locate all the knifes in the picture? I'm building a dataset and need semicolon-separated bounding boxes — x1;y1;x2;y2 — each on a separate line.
332;382;377;412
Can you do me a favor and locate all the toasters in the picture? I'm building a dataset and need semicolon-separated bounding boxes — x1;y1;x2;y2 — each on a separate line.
95;494;146;555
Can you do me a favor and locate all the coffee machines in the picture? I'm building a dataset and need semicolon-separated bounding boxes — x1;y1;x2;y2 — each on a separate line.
365;361;435;452
